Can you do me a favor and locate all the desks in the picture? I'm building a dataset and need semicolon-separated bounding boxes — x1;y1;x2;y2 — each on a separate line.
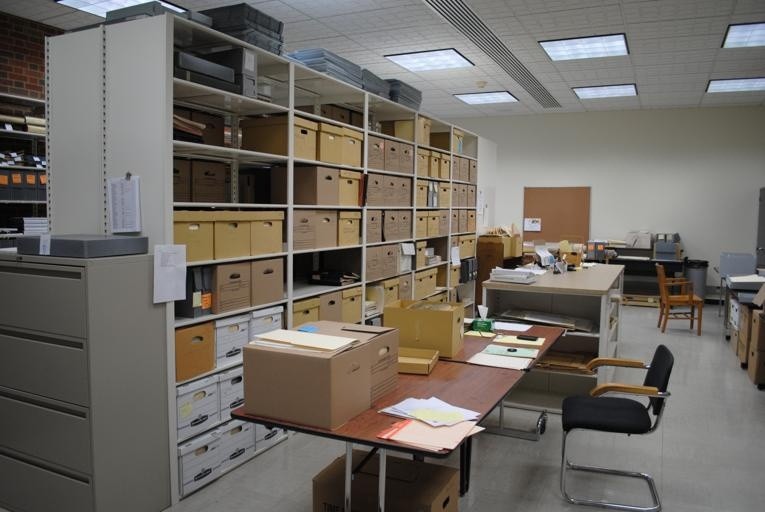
230;315;565;510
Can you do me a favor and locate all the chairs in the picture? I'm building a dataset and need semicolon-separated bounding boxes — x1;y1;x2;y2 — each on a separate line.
655;262;703;336
561;341;675;511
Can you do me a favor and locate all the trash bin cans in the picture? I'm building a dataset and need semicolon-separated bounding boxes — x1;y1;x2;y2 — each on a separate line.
685;260;709;299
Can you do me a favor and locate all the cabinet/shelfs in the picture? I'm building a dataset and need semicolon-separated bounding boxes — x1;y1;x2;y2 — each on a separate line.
1;92;45;246
43;11;499;506
0;249;170;511
604;256;689;307
481;261;626;416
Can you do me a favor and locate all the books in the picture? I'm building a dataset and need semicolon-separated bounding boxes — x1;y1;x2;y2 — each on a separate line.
489;268;537;285
249;327;361;363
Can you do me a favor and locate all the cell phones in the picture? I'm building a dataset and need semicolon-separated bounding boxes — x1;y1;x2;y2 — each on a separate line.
517;335;538;340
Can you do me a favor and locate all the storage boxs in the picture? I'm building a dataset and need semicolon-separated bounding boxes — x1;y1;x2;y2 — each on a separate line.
719;251;757;279
476;227;523;307
727;294;765;385
